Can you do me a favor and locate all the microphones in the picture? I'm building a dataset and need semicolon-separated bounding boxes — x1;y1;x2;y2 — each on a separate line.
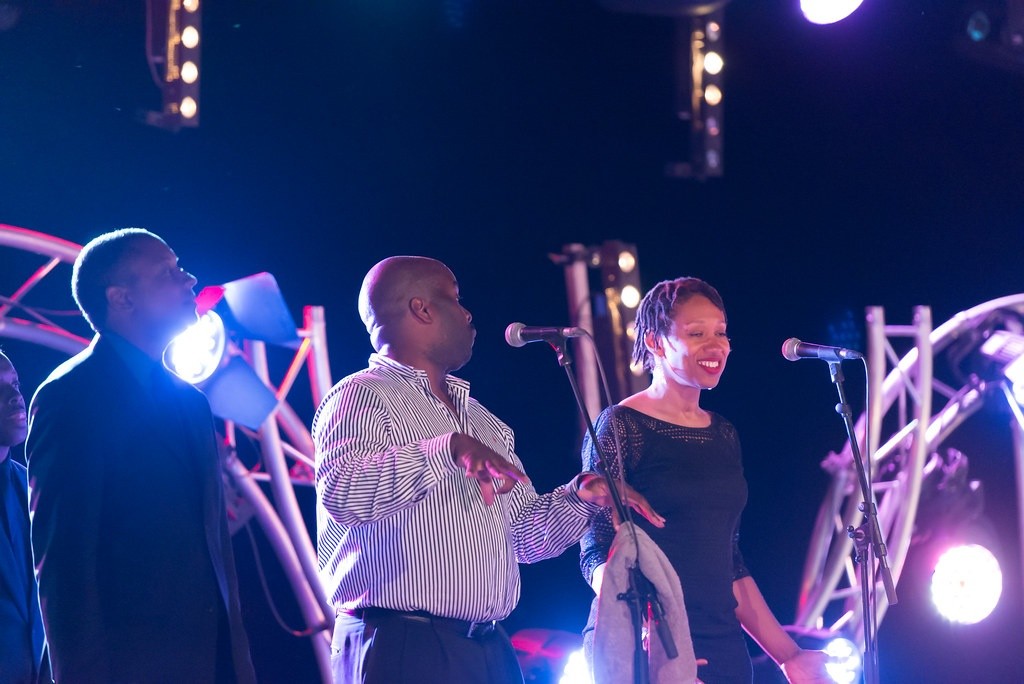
505;322;586;348
782;338;863;361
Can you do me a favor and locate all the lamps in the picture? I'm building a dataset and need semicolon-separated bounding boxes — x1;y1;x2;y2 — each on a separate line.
767;626;865;684
598;242;658;402
147;0;203;131
923;512;1007;627
688;1;729;177
159;270;301;432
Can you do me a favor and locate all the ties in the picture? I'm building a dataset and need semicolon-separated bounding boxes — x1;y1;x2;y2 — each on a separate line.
3;466;31;609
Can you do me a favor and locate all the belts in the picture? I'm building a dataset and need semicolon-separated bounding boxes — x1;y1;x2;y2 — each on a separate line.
356;606;497;645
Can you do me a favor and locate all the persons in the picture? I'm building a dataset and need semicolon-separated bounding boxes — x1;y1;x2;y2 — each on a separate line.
308;255;667;684
0;351;48;684
22;224;260;683
578;277;850;683
